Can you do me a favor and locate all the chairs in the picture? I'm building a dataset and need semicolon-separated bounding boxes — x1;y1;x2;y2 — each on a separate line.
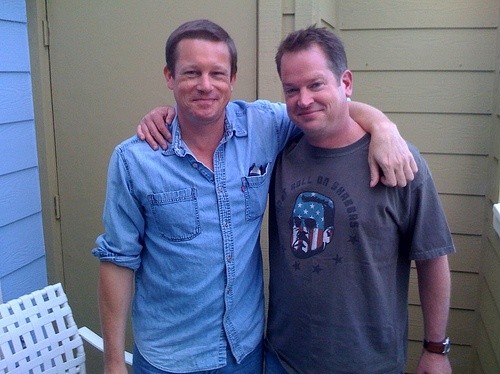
0;282;134;374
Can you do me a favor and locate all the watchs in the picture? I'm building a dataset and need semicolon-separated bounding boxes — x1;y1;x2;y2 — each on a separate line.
422;336;451;354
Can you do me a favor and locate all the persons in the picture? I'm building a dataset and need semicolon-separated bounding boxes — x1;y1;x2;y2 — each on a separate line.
91;19;420;374
135;23;454;374
